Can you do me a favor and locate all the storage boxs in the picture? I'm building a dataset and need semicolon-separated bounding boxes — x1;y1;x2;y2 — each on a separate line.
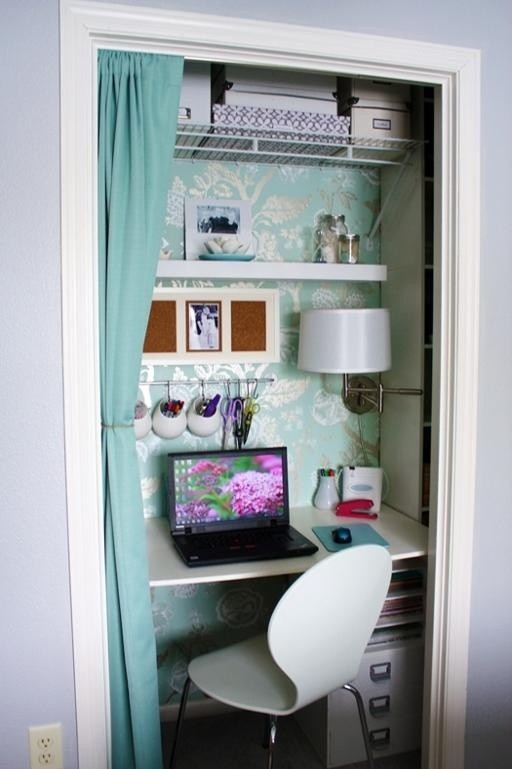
175;61;411;165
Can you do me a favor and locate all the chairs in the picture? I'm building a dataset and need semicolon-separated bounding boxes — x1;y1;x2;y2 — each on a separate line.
169;544;392;769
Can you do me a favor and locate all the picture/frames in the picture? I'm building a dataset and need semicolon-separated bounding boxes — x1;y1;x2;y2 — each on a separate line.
142;288;280;365
184;198;252;260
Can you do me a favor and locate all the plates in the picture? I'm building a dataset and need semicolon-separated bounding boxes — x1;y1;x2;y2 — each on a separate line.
198;253;254;262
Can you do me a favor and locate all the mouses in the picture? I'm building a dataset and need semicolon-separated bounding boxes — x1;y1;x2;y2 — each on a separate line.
332;526;353;544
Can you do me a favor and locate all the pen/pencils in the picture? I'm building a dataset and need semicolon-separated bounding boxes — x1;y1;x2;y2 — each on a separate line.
320;468;334;476
198;394;221;417
163;399;184;418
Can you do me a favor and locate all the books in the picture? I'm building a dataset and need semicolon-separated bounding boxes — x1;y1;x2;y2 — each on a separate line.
365;570;427;651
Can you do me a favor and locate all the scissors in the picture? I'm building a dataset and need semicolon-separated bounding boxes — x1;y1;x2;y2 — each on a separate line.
220;397;260;449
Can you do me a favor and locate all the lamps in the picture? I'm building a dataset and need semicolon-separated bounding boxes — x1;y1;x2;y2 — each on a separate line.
297;308;423;415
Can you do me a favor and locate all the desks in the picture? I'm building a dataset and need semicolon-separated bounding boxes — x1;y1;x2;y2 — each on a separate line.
146;503;429;769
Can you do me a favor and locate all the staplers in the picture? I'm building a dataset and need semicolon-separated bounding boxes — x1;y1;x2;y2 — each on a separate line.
336;499;377;519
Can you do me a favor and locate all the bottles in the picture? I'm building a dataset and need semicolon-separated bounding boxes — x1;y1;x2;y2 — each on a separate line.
338;233;361;264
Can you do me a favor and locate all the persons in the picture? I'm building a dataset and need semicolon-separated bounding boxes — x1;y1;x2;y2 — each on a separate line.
195;305;217;349
198;206;239;234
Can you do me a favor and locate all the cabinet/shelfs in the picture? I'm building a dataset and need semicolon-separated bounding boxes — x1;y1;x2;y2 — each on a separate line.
157;121;430;281
379;87;432;523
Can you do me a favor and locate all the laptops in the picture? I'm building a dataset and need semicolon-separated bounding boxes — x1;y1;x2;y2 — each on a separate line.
167;446;318;568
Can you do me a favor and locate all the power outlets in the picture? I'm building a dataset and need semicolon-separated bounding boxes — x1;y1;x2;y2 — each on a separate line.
29;724;64;769
